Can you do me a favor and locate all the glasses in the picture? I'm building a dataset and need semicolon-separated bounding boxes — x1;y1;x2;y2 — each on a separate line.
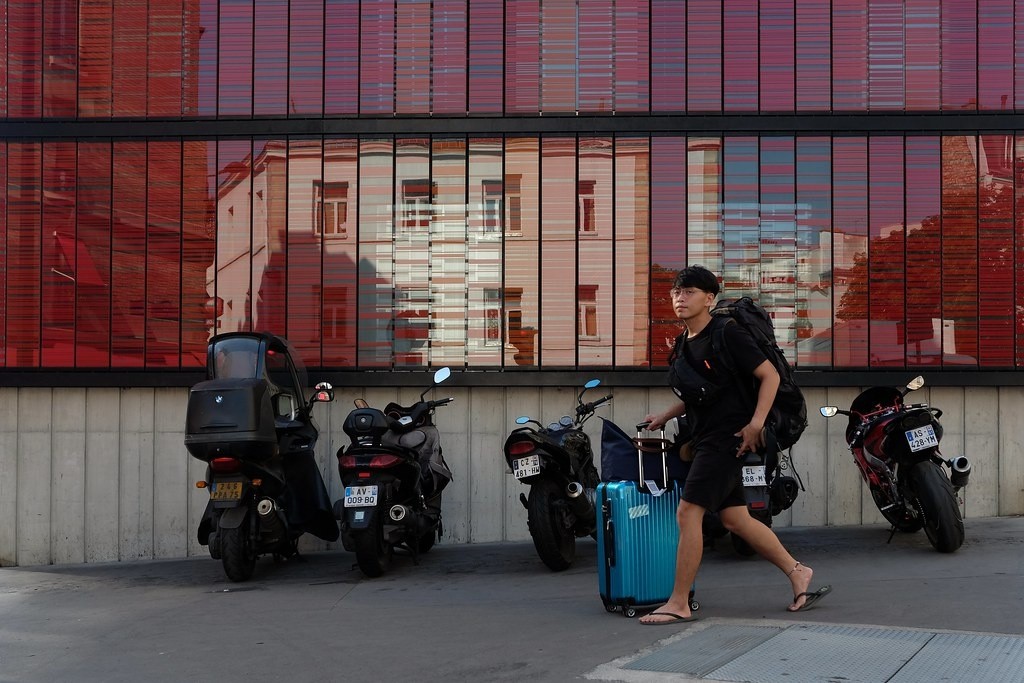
670;288;702;300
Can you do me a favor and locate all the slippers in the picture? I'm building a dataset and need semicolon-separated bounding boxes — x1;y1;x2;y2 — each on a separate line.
787;586;831;611
640;612;698;624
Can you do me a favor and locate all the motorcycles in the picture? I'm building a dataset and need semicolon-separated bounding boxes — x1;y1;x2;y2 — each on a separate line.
503;379;615;573
332;367;455;578
183;331;340;582
820;375;973;553
672;414;799;556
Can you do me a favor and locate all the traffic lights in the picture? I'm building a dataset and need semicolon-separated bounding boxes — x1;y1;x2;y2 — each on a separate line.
513;327;535;365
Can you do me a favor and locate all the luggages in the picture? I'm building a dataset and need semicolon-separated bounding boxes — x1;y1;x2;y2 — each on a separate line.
594;422;699;617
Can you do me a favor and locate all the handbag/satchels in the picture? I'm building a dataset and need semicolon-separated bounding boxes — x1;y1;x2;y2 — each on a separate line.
666;333;720;410
600;418;692;480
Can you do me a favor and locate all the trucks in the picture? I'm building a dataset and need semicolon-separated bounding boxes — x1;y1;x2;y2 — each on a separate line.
782;319;898;367
257;250;424;368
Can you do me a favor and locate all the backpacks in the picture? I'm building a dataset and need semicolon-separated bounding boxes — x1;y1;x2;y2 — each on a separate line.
681;297;805;448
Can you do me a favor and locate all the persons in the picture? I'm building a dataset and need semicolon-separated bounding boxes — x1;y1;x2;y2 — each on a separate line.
638;265;833;625
